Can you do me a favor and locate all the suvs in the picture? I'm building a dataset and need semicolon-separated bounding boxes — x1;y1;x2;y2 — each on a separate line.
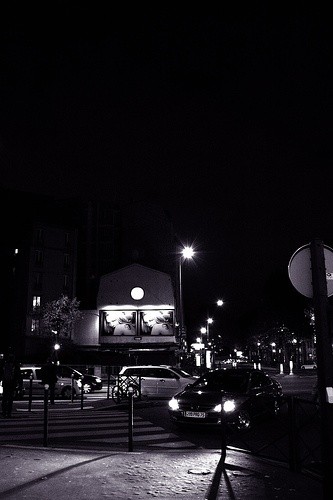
33;364;103;394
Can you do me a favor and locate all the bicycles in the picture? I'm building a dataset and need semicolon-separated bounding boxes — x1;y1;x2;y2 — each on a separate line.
110;374;142;403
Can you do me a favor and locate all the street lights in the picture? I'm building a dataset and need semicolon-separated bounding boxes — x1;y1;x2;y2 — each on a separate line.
179;243;196;339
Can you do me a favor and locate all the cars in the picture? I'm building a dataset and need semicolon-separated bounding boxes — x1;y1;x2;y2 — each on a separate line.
119;365;208;399
301;362;317;370
18;367;79;400
167;369;284;435
